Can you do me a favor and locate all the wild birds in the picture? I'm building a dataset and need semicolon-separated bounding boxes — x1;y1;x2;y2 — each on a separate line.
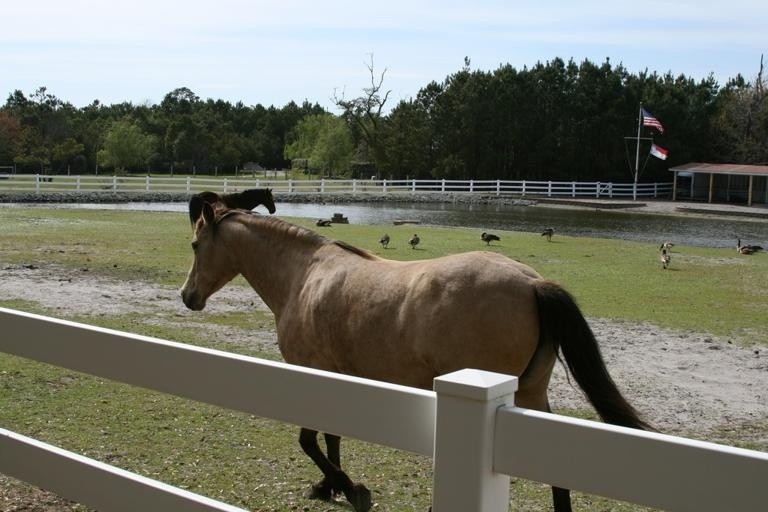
481;233;500;246
660;242;674;254
737;239;763;254
541;225;553;241
317;219;331;227
661;250;670;269
408;234;419;249
380;234;389;249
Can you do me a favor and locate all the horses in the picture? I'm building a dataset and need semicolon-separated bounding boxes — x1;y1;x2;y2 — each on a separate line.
178;202;663;512
189;188;276;235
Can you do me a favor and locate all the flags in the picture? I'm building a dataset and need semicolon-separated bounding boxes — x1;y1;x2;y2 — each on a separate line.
640;108;664;136
650;143;668;162
